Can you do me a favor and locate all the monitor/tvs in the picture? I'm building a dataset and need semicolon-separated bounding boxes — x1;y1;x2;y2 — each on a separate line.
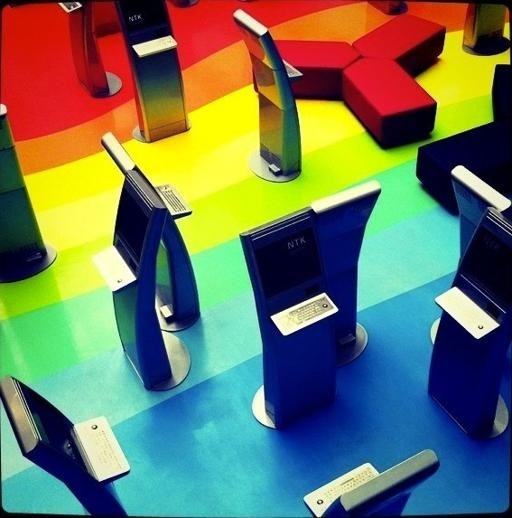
461;227;512;307
253;227;320;299
116;189;148;266
17;378;84;466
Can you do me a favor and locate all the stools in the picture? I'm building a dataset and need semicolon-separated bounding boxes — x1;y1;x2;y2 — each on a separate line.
352;14;445;77
342;58;436;148
274;41;361;101
